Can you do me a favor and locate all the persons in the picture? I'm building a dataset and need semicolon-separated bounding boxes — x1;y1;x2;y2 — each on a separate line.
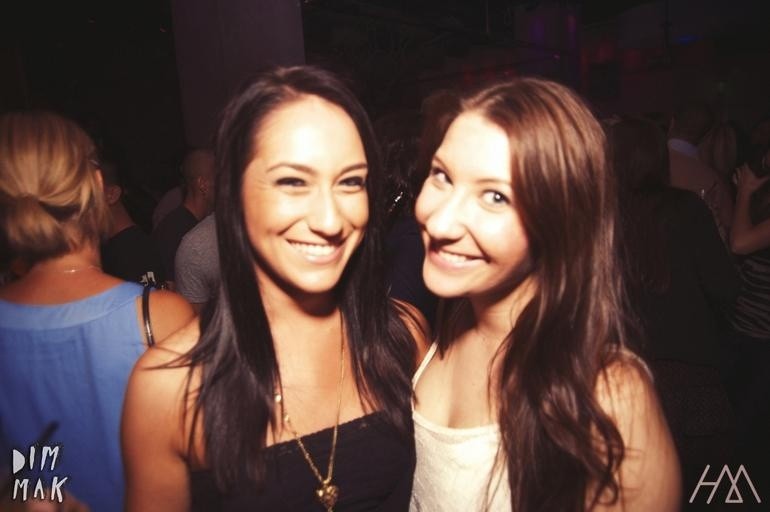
0;105;199;511
90;100;769;511
122;63;432;511
405;74;684;511
729;146;769;510
